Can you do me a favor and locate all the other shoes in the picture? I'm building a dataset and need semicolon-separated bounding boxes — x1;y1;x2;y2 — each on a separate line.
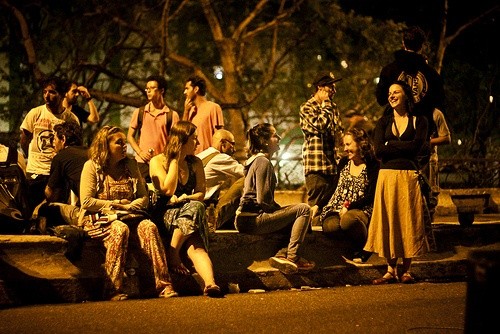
352;250;372;264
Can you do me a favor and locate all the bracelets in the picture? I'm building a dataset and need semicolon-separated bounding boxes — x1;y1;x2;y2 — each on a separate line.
86;96;92;101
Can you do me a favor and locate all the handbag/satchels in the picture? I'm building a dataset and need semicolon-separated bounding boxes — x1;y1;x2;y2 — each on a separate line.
429;189;440;208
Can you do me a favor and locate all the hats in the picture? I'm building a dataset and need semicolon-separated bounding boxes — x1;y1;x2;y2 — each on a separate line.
313;75;342;87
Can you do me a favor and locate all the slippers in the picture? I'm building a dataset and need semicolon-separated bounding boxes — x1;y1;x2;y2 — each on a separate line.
204;284;220;296
170;264;190;275
294;257;316;271
269;256;298;274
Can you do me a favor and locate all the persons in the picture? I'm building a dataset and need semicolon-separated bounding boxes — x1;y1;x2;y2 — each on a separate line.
235;123;316;274
61;79;99;124
300;76;344;226
183;75;225;155
320;128;379;265
149;120;221;294
425;109;451;207
195;129;246;229
362;80;436;285
375;26;443;153
126;74;180;184
33;122;89;235
79;125;177;300
20;77;80;209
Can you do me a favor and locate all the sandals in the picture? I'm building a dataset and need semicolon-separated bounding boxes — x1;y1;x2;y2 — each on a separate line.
109;293;128;301
401;273;411;283
157;287;177;297
373;272;399;284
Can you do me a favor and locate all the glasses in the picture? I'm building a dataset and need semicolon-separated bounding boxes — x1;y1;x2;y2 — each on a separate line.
145;87;162;91
225;139;236;146
108;126;122;135
